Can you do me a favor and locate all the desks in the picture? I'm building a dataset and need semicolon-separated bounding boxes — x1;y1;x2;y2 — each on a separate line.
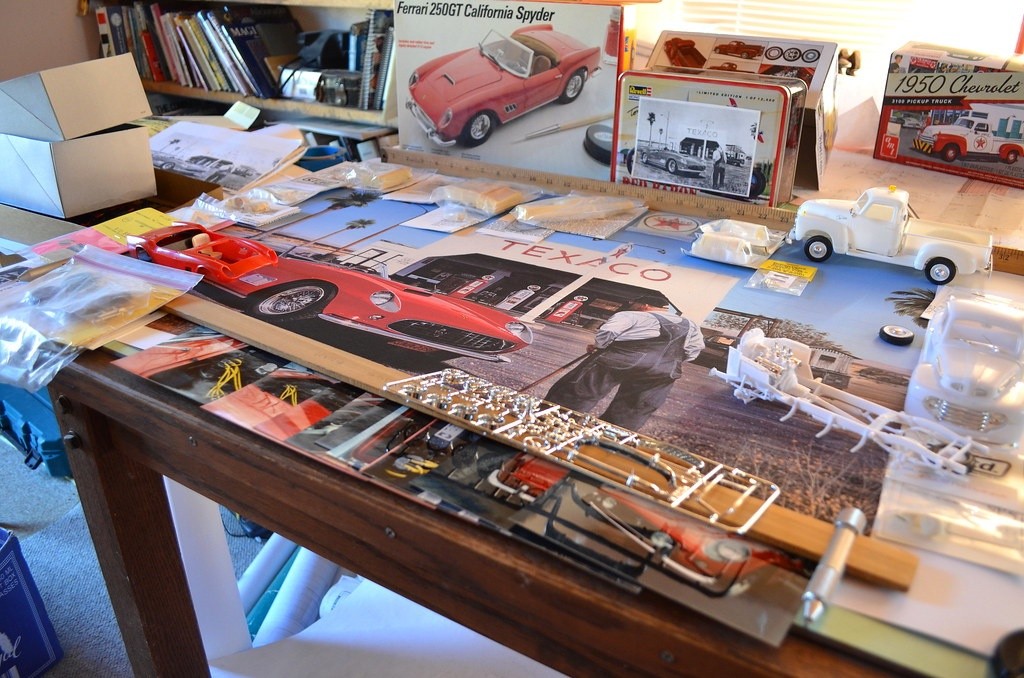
46;349;927;678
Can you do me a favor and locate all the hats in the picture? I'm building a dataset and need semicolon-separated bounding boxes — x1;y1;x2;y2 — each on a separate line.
634;295;669;307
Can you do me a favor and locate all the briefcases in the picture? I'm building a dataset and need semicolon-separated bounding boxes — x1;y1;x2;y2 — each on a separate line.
0;383;74;479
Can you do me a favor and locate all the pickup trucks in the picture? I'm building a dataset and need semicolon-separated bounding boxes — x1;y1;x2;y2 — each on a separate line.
909;115;1024;165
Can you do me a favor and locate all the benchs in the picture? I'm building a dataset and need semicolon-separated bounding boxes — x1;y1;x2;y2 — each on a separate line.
944;322;1018;351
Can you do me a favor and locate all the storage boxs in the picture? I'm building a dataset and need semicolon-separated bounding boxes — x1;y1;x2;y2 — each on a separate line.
610;64;808;209
0;53;153;142
644;29;839;191
872;40;1024;190
0;168;223;245
0;124;158;219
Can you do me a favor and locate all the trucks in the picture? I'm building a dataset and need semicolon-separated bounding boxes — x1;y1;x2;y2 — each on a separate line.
790;184;994;286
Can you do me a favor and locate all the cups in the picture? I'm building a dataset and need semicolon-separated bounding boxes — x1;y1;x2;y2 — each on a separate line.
298;146;345;172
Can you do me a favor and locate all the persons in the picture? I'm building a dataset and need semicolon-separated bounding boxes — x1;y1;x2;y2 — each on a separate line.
538;295;706;433
890;55;903;73
712;144;726;189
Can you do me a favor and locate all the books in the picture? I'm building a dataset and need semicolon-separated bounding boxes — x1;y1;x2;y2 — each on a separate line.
96;2;397;121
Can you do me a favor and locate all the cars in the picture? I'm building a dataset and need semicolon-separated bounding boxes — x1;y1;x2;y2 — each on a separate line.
129;219;535;363
404;25;602;151
642;147;706;179
